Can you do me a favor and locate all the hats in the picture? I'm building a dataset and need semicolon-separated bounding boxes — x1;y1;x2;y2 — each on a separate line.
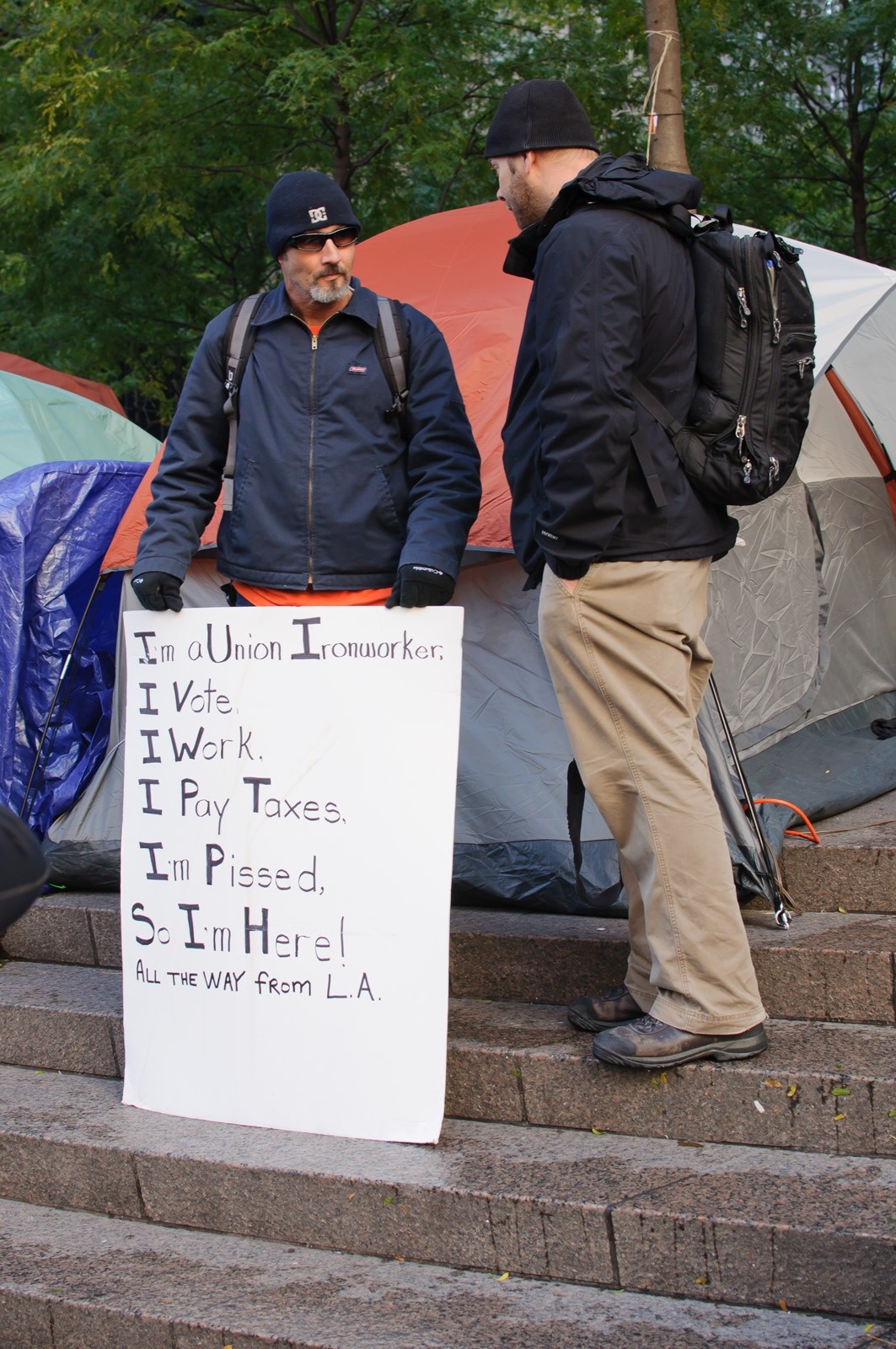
266;171;362;259
484;81;599;158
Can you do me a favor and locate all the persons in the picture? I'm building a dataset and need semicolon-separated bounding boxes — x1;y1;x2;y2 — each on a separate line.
487;79;769;1071
132;171;480;610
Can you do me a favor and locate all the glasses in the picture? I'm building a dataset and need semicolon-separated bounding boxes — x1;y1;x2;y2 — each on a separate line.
288;226;360;251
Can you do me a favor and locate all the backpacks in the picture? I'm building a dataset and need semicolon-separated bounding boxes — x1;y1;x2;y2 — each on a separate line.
586;196;816;505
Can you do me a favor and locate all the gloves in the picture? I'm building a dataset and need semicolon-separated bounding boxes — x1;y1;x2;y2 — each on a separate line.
387;564;454;608
131;572;184;612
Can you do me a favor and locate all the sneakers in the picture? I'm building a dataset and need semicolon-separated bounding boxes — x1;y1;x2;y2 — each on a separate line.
592;1015;766;1068
568;986;639;1035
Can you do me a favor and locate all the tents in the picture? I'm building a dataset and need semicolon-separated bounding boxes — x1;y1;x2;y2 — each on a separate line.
2;351;168;867
89;188;896;925
